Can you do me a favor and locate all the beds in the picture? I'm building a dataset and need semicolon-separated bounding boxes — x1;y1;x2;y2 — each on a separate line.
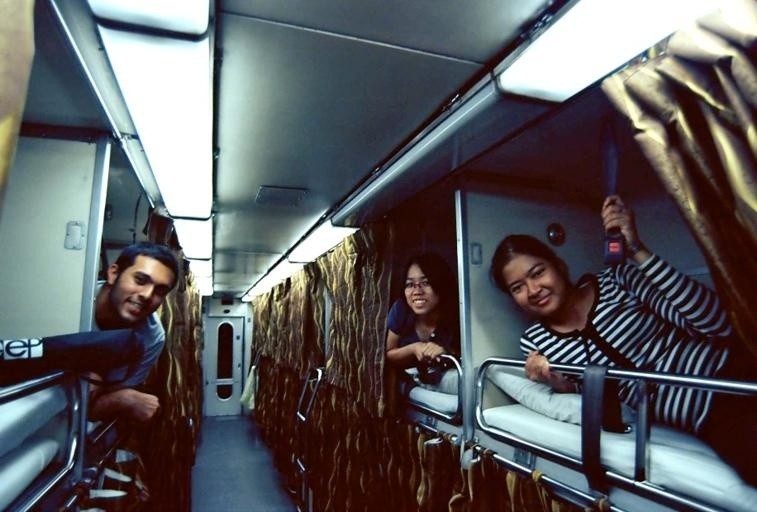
0;349;133;511
468;352;757;512
388;353;464;440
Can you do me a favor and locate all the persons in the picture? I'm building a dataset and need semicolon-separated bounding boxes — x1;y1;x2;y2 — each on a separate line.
487;191;755;491
384;257;459;362
88;240;178;422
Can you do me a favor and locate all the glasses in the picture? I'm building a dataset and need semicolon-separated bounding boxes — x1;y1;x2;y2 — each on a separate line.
404;281;431;290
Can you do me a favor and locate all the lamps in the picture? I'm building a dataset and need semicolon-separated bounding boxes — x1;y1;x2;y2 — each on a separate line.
243;258;307;302
87;0;216;299
491;1;731;103
325;73;552;224
286;220;359;264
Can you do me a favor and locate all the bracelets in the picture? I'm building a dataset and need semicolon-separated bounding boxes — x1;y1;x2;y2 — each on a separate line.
627;239;643;258
